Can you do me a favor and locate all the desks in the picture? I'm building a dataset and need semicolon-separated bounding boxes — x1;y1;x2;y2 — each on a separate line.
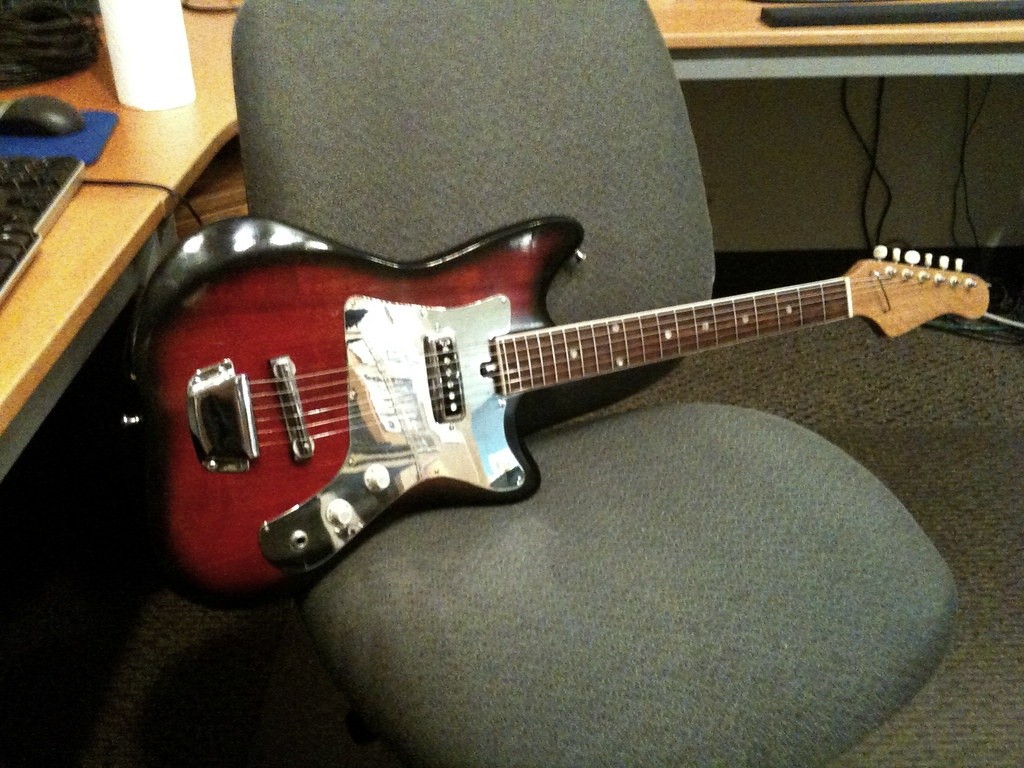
648;0;1024;123
0;2;241;495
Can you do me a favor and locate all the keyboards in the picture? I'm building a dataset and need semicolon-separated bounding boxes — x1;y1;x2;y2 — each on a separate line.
0;156;86;308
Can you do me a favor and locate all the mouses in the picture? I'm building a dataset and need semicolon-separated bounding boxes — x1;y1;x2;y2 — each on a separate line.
0;94;85;136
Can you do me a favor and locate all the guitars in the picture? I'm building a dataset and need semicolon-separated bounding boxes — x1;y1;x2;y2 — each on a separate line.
122;214;991;610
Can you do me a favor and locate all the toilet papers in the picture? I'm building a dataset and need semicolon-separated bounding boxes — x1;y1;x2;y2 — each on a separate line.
98;0;197;112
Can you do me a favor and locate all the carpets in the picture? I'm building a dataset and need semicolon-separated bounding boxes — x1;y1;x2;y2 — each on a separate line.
0;279;1024;768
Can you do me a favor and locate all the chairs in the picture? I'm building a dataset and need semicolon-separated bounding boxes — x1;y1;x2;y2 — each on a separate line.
230;0;960;768
